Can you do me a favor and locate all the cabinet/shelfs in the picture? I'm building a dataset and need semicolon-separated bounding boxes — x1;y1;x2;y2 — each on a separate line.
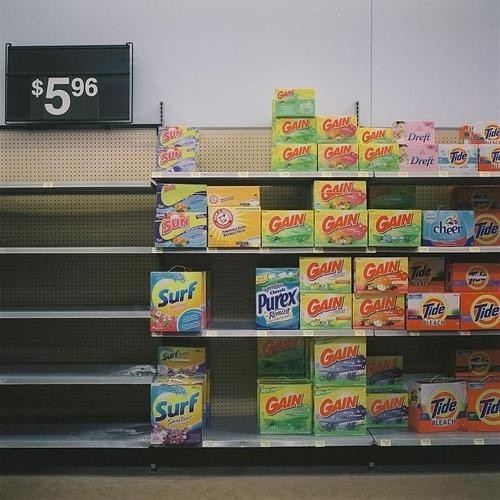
151;127;500;473
0;124;160;470
